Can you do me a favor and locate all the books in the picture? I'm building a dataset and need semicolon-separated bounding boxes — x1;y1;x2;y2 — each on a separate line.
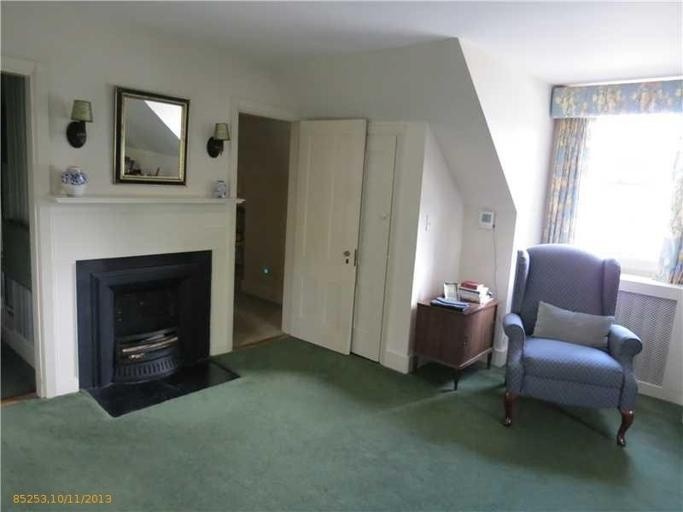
458;280;488;305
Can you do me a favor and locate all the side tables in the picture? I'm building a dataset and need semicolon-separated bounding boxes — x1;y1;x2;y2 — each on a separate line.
415;295;497;390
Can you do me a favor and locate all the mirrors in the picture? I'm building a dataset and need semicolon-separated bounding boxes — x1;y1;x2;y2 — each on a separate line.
112;85;191;184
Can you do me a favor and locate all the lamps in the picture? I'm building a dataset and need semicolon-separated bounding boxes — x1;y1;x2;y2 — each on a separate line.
66;98;95;149
206;121;229;158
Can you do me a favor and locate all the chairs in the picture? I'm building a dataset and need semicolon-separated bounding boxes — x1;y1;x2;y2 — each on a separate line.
501;241;642;446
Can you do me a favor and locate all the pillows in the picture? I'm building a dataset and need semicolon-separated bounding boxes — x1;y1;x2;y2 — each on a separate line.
525;295;616;348
